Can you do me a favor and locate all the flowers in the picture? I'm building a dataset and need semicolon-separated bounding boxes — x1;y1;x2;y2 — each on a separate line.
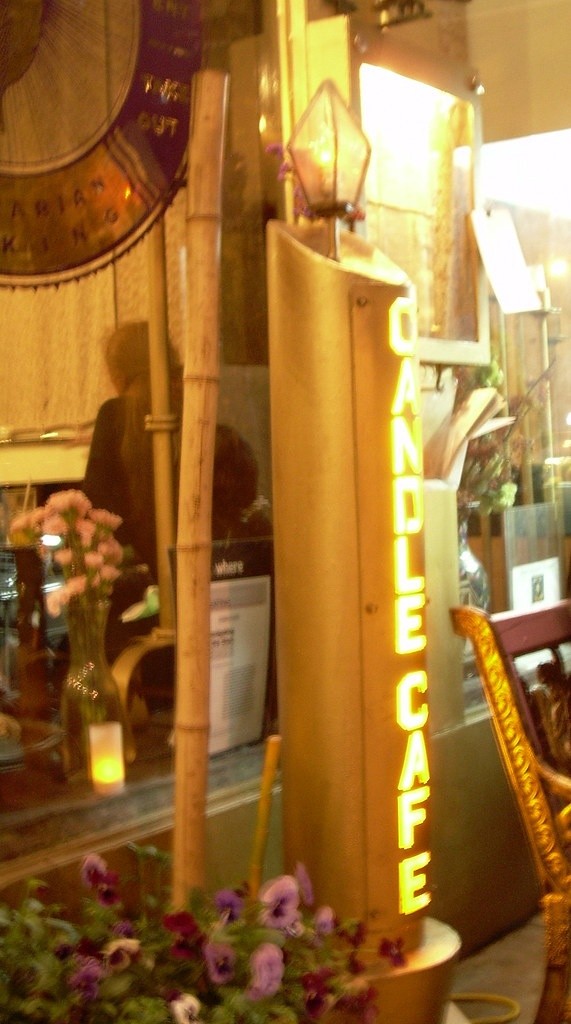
12;489;126;618
1;836;412;1023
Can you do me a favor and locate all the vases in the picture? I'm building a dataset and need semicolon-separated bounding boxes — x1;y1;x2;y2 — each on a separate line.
64;602;135;784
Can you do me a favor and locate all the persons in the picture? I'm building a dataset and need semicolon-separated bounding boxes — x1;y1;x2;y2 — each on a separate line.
78;321;258;717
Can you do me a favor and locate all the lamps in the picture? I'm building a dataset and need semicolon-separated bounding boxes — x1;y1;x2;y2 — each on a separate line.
287;80;372;256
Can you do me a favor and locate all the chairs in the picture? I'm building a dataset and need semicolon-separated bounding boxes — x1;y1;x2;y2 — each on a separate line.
449;598;571;1024
